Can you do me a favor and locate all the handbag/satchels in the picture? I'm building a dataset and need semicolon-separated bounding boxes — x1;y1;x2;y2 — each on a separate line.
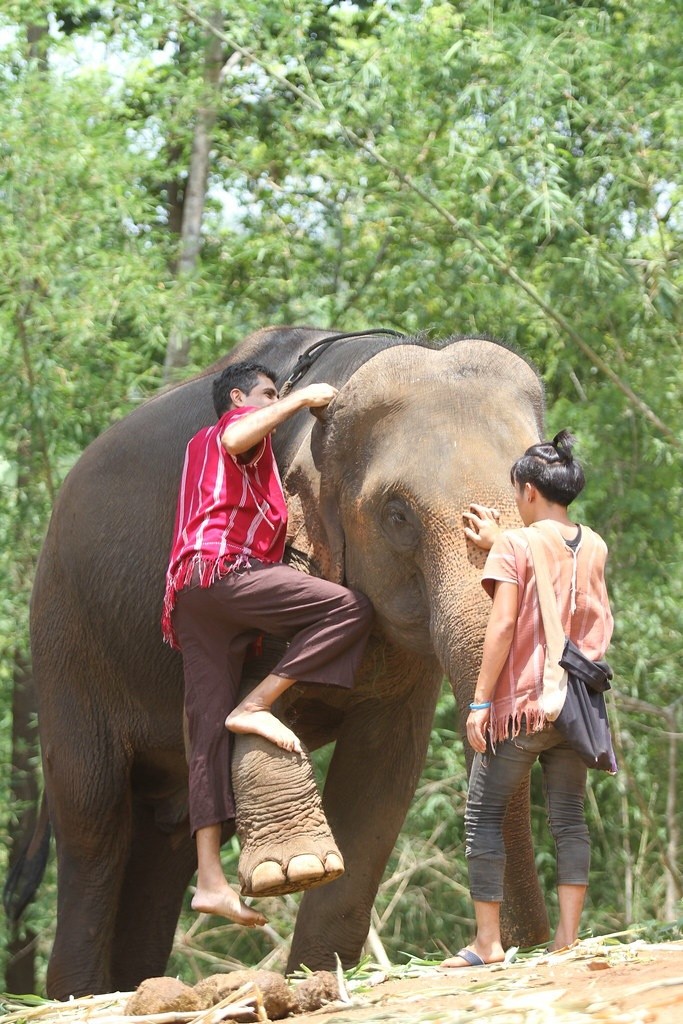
543;638;618;773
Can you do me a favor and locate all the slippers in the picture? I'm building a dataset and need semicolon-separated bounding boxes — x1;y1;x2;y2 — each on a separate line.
440;946;504;968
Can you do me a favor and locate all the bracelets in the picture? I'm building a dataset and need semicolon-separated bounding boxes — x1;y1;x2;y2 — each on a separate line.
468;701;492;710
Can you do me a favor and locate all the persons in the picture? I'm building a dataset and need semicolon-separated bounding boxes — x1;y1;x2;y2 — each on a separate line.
161;361;376;929
438;424;614;969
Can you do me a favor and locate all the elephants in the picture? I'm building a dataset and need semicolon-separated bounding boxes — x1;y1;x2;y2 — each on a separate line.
2;320;573;1009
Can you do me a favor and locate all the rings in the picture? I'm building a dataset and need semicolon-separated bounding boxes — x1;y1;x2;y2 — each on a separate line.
493;516;501;520
